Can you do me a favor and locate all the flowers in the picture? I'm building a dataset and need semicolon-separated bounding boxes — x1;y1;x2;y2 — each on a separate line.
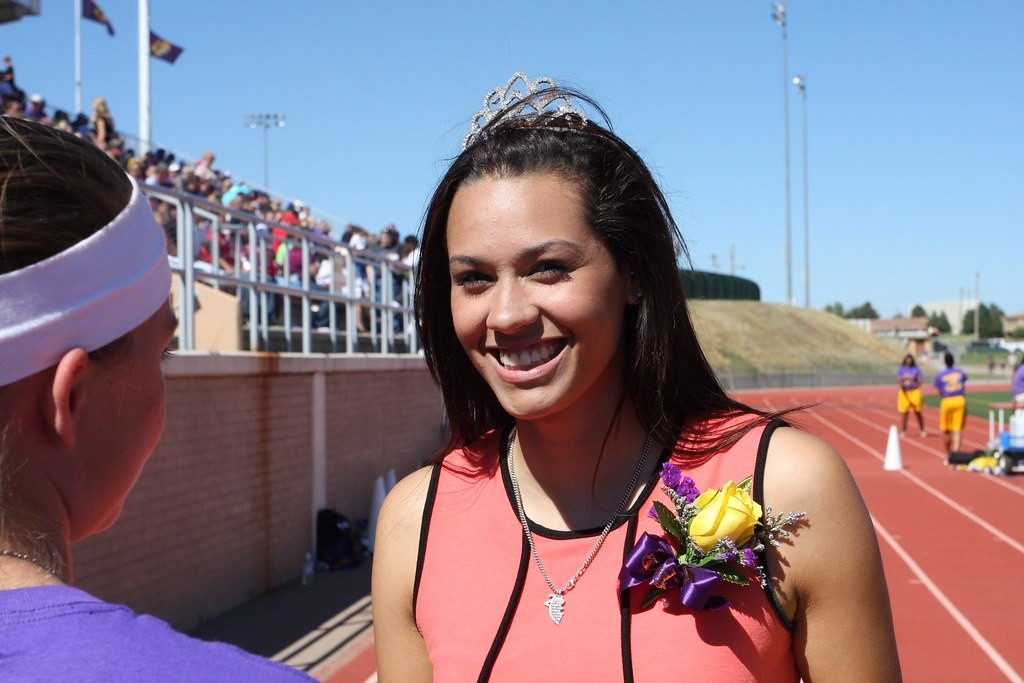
646;461;807;590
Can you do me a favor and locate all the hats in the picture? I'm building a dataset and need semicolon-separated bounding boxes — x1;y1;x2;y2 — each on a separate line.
168;163;180;170
29;94;46;103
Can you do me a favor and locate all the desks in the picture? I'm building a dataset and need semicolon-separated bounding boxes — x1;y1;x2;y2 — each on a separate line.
988;402;1024;440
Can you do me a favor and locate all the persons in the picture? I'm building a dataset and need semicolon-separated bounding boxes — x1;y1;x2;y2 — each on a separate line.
1009;349;1024;414
897;354;928;438
371;70;902;683
932;353;967;466
0;117;318;683
0;55;419;334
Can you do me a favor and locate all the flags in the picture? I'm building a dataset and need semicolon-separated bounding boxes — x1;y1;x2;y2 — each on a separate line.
150;31;182;64
80;0;114;36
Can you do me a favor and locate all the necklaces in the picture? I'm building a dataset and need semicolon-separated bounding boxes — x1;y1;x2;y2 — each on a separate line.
0;549;68;584
509;424;654;628
